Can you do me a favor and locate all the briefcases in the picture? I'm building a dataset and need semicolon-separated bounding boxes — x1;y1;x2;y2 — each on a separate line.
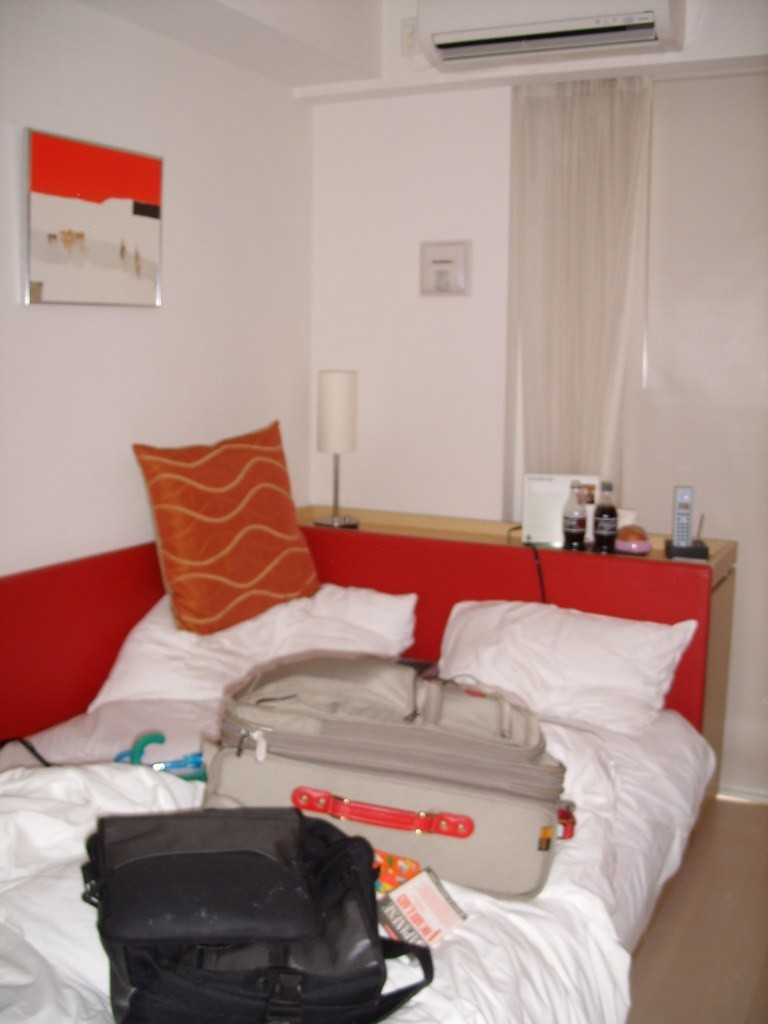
201;649;576;898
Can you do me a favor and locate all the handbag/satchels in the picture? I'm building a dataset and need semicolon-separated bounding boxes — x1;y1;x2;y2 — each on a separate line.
80;805;436;1024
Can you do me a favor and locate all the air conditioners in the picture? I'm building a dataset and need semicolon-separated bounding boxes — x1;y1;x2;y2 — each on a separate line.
414;0;683;72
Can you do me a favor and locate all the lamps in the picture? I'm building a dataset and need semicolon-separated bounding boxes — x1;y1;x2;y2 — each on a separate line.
314;370;359;531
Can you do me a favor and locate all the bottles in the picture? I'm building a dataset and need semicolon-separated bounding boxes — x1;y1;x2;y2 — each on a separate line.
591;483;618;554
561;479;587;552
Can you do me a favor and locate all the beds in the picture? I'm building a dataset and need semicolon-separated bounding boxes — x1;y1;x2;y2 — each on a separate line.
0;529;716;1024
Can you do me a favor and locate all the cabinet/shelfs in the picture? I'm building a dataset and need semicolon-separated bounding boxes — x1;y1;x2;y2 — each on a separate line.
510;527;738;798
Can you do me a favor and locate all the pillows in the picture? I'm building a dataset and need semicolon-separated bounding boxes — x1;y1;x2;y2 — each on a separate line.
132;422;319;635
438;599;697;738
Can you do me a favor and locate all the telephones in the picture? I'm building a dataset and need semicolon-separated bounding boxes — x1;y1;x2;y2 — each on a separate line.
664;485;709;560
